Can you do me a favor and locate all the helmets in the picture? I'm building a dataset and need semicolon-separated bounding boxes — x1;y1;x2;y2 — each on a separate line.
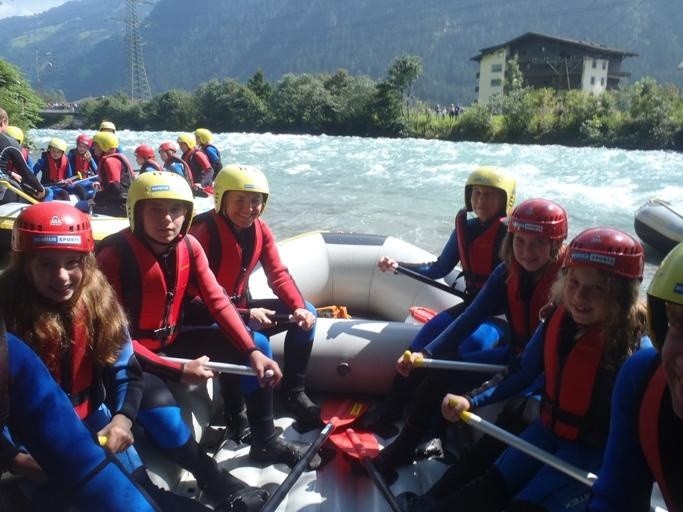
10;202;95;254
47;121;211;159
6;125;24;146
464;167;681;351
213;164;269;216
125;171;194;235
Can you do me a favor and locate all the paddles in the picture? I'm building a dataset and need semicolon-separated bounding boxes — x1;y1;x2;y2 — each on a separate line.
328;423;402;510
258;395;373;512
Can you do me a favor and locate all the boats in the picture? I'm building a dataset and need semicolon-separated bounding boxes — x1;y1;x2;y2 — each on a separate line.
0;169;216;259
141;229;468;512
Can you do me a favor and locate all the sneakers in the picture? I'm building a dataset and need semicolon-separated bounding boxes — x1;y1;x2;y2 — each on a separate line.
397;490;442;512
223;408;252;442
250;440;310;464
358;394;389;429
282;391;320;423
352;437;415;476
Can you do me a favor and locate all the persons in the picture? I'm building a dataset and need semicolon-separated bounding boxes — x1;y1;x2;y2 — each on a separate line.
0;201;215;512
434;102;461;121
352;198;569;495
398;228;649;511
0;315;161;512
95;168;337;508
582;245;683;511
0;108;224;218
356;167;518;428
188;164;322;440
